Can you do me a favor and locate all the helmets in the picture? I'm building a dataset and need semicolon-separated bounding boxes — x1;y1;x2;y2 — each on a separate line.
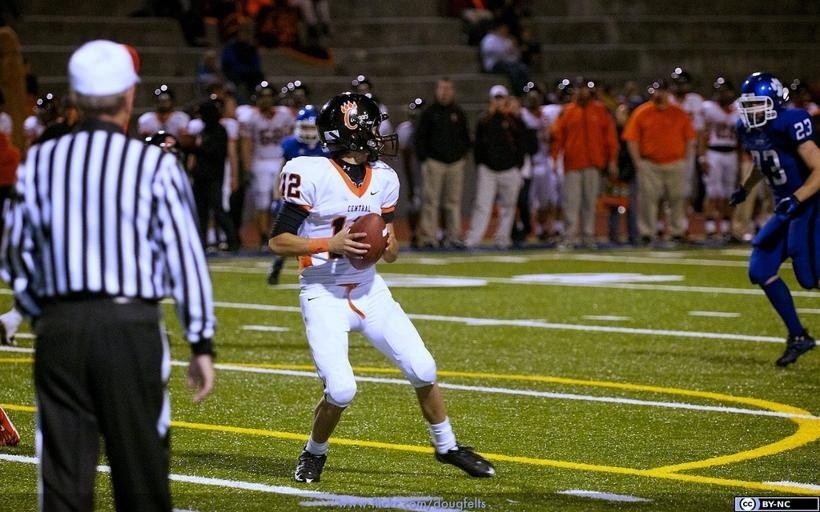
734;72;786;129
295;106;318;144
316;92;399;157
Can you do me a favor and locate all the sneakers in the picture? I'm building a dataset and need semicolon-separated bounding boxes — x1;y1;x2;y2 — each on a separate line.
776;329;815;368
433;446;495;478
294;445;327;483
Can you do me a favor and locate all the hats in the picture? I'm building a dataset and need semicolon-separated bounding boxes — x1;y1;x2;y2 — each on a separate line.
488;84;510;99
68;40;142;96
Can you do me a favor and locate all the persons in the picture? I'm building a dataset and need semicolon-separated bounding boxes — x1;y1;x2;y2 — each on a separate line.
267;104;331;287
0;0;820;252
732;68;820;368
0;39;218;512
265;92;496;482
0;302;25;344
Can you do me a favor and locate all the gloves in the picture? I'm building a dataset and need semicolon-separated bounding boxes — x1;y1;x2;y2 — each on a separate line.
729;186;749;207
773;192;802;218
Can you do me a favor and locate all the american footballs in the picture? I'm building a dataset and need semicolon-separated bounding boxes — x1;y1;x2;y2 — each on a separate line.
349;213;388;269
